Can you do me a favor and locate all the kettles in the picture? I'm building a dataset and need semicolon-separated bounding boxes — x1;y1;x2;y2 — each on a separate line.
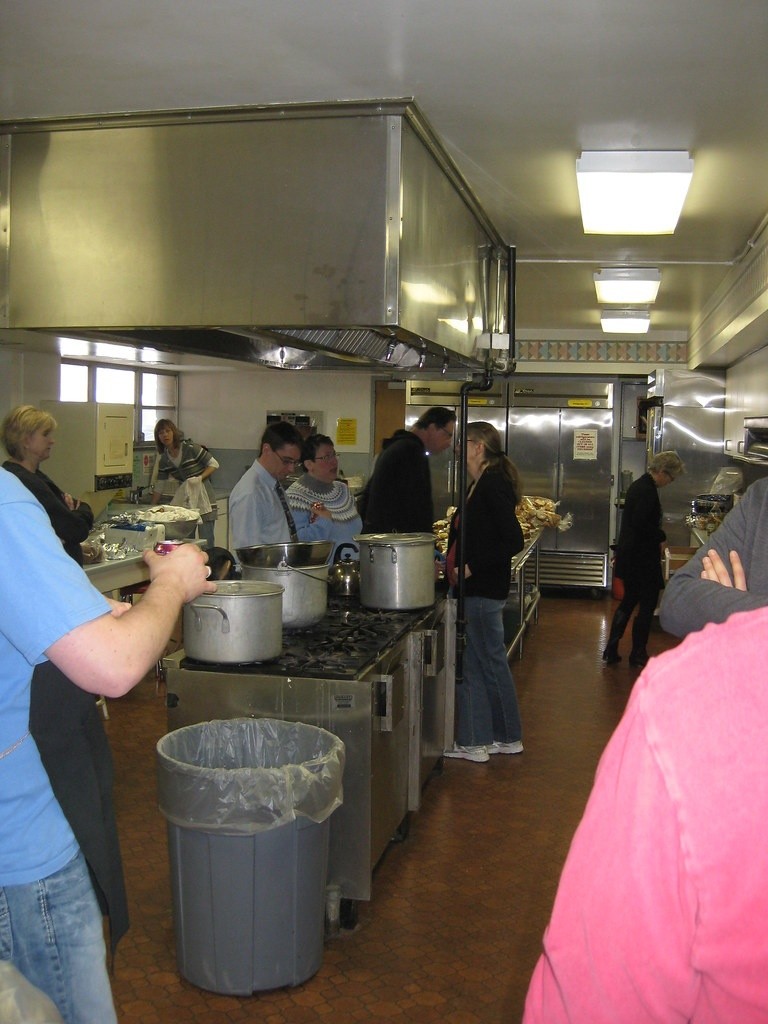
329;542;361;596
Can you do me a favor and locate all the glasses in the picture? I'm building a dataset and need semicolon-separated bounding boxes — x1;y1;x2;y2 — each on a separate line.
439;426;452;439
270;448;303;468
457;439;474;446
311;451;342;463
665;472;675;482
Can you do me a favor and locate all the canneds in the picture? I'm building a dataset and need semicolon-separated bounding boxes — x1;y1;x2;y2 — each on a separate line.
153;539;184;555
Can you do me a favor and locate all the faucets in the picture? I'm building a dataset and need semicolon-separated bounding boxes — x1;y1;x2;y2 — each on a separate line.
130;484;154;502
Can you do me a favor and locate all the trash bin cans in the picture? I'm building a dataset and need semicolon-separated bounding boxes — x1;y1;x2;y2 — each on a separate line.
156;719;347;996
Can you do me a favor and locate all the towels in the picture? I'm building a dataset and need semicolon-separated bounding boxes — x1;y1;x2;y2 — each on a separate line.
169;475;212;525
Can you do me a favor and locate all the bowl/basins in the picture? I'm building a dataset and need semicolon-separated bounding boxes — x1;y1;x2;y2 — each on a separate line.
347;486;363;496
232;539;336;567
136;515;200;540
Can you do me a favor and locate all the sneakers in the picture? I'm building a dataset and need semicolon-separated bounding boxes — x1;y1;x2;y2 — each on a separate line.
487;738;524;755
444;741;490;762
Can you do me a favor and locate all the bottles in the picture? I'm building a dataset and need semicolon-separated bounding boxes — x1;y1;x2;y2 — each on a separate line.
707;502;721;536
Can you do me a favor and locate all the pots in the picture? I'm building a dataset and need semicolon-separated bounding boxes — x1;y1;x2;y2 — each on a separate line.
182;580;286;664
352;532;439;609
233;562;331;628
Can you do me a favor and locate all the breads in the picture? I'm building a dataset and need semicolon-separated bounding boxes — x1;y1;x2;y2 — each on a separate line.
435;497;563;552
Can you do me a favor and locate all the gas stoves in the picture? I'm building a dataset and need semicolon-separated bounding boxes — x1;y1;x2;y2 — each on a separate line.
180;594;447;680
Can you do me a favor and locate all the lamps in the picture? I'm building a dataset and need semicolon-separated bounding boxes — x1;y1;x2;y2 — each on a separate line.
575;151;695;235
594;268;662;304
600;309;650;333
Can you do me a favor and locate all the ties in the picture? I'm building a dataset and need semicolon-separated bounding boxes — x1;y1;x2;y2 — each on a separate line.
276;481;297;540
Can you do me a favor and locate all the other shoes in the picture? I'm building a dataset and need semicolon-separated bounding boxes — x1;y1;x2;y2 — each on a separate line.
628;652;650;666
603;650;622;662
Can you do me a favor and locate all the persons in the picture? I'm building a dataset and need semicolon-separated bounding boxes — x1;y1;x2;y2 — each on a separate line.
151;418;220;551
659;476;768;638
444;422;524;762
603;451;685;666
521;607;768;1024
286;433;364;564
0;467;218;1024
0;404;94;567
229;421;305;570
360;407;456;534
